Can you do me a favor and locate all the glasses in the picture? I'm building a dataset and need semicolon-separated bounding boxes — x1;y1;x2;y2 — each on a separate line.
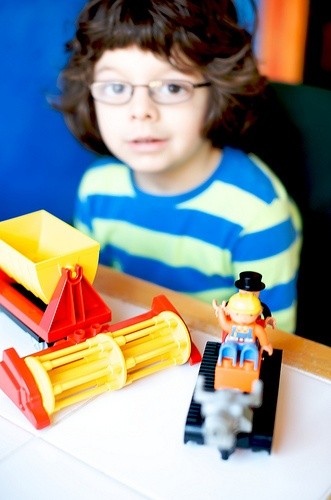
88;79;213;105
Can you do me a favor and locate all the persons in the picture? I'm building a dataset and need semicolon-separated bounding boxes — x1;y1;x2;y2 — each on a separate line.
213;293;273;371
225;271;275;350
46;0;301;334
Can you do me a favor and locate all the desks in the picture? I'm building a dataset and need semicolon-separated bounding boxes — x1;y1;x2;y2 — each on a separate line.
0;261;331;500
